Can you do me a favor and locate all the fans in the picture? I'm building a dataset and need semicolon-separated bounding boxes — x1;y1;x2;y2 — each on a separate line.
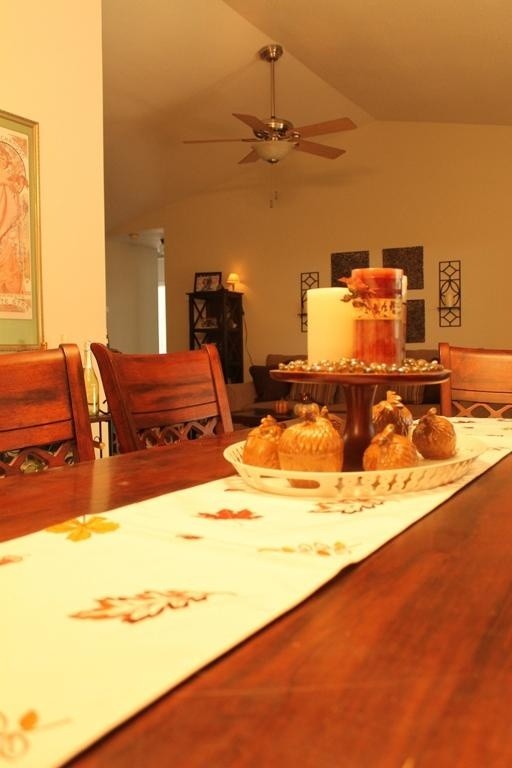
182;44;357;164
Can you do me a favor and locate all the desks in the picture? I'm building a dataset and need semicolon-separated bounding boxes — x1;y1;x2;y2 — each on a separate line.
0;411;512;767
90;414;115;456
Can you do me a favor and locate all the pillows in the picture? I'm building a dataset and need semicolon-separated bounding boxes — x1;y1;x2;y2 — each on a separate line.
390;384;425;404
290;383;338;406
338;384;384;405
248;360;292;402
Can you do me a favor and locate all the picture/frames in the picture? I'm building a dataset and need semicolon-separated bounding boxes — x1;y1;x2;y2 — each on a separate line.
194;272;222;292
0;109;45;351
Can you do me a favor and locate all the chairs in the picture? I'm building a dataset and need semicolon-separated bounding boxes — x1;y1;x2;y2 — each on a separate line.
0;344;96;476
91;342;234;454
439;342;512;418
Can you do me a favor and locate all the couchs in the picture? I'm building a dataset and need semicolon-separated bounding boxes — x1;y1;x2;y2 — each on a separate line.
225;350;441;427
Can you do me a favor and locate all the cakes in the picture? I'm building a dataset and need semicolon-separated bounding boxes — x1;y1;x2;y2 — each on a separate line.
238;389;459;473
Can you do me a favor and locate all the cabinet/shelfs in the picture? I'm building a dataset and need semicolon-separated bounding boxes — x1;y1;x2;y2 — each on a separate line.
185;291;243;384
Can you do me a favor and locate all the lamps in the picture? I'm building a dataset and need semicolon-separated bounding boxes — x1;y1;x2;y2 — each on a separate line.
226;273;240;291
251;135;298;164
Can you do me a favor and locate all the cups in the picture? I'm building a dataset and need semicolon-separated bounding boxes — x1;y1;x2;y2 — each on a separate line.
351;268;409;368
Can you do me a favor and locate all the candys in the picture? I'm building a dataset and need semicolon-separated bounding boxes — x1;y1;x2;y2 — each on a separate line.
278;357;444;373
